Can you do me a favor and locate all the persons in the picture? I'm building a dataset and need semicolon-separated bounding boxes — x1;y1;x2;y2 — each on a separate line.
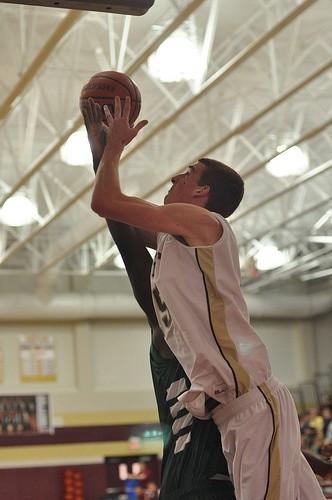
90;95;326;500
298;403;332;478
81;98;236;500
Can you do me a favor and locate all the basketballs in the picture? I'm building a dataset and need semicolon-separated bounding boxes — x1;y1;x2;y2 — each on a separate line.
80;70;142;127
